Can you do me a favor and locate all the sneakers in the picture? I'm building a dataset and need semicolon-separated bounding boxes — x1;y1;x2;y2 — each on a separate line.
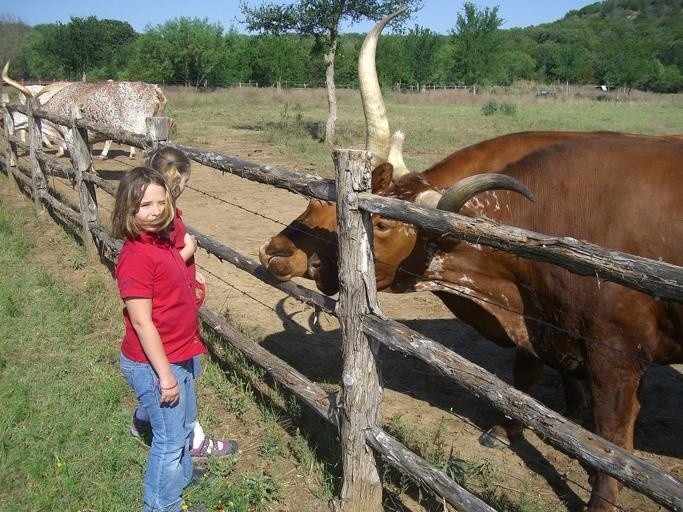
131;408;151;437
180;505;207;512
191;468;216;485
190;436;233;460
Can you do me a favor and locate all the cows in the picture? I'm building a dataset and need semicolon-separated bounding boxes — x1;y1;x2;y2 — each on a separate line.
0;56;167;162
258;3;681;512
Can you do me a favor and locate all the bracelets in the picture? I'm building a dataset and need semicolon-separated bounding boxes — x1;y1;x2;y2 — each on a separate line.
154;377;178;389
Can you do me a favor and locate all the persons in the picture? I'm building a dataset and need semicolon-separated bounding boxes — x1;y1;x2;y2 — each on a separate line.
129;145;240;459
106;165;213;511
